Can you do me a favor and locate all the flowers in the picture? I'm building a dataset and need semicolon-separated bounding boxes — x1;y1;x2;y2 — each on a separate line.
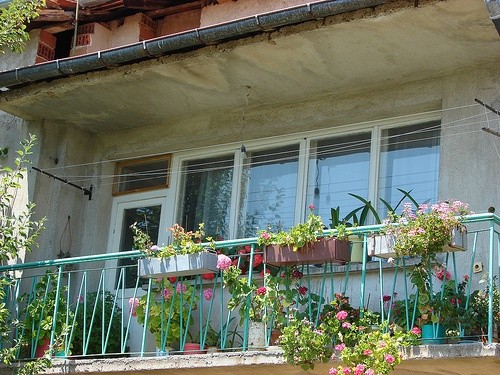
128;200;500;375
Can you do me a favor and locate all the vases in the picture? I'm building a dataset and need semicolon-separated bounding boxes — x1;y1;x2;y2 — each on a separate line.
270;329;283;345
137;251;218;279
367;225;467;258
263;239;352;268
156;346;175;356
423;325;446;345
247;320;266;352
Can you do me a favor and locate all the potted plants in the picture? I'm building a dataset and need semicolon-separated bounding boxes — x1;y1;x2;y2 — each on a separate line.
17;269;78;358
328;200;372;263
170;320;212;355
202;317;244;354
